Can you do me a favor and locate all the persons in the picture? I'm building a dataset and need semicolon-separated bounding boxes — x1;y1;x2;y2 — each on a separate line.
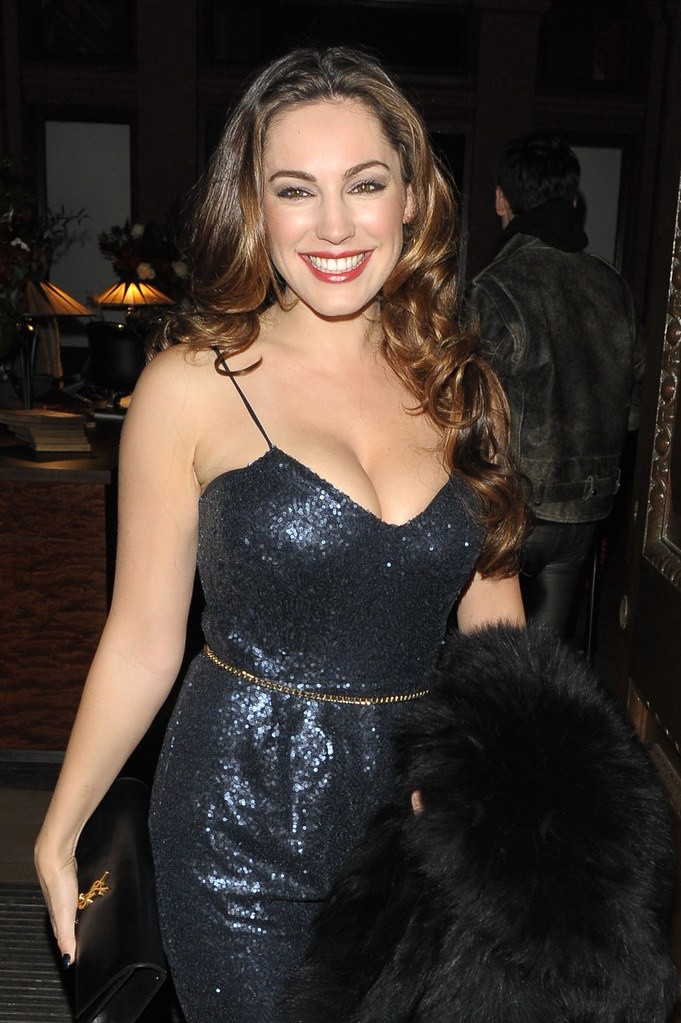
460;139;648;637
32;45;525;1023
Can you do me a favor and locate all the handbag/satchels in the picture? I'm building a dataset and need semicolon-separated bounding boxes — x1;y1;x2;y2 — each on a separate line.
44;778;166;1023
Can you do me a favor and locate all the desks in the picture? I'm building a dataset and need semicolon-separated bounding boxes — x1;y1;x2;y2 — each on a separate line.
0;443;117;612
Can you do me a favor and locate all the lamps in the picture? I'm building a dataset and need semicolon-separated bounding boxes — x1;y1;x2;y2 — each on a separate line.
13;282;96;410
87;279;177;321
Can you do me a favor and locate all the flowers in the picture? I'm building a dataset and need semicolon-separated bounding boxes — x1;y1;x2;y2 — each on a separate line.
96;218;190;284
0;184;95;360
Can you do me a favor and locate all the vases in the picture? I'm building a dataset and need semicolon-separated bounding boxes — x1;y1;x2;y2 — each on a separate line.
85;321;143;392
